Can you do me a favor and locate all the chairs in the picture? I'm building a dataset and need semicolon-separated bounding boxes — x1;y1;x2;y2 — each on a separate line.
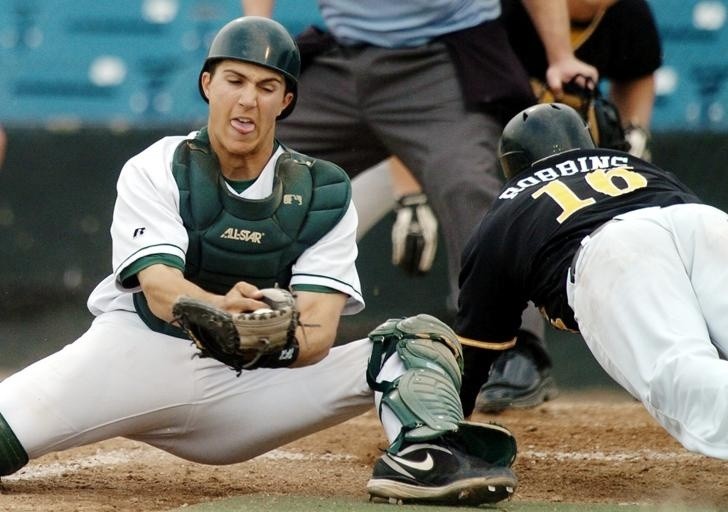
2;2;723;139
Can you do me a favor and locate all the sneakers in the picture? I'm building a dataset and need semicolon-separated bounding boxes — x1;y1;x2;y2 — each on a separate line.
367;432;517;504
476;351;559;413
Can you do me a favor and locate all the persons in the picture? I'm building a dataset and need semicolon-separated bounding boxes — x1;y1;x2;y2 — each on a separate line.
0;15;518;505
243;1;600;414
470;0;662;163
448;103;728;470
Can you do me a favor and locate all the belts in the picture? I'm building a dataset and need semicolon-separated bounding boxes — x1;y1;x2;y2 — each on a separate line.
569;217;622;284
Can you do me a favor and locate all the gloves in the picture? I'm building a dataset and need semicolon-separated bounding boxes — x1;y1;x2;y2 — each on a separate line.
389;191;440;275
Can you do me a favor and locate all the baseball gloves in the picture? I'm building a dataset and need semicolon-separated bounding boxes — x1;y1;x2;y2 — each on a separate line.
171;287;297;371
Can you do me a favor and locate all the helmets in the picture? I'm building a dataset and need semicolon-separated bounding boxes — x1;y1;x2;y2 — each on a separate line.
496;103;598;180
198;16;301;122
